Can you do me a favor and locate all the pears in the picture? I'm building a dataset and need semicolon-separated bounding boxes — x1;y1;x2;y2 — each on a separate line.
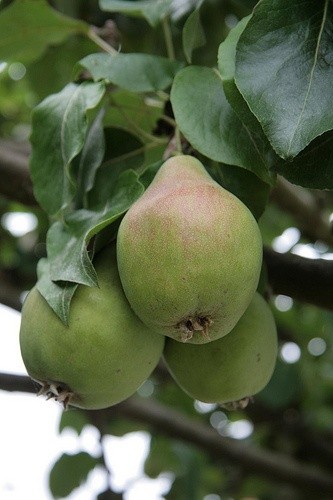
19;155;278;412
